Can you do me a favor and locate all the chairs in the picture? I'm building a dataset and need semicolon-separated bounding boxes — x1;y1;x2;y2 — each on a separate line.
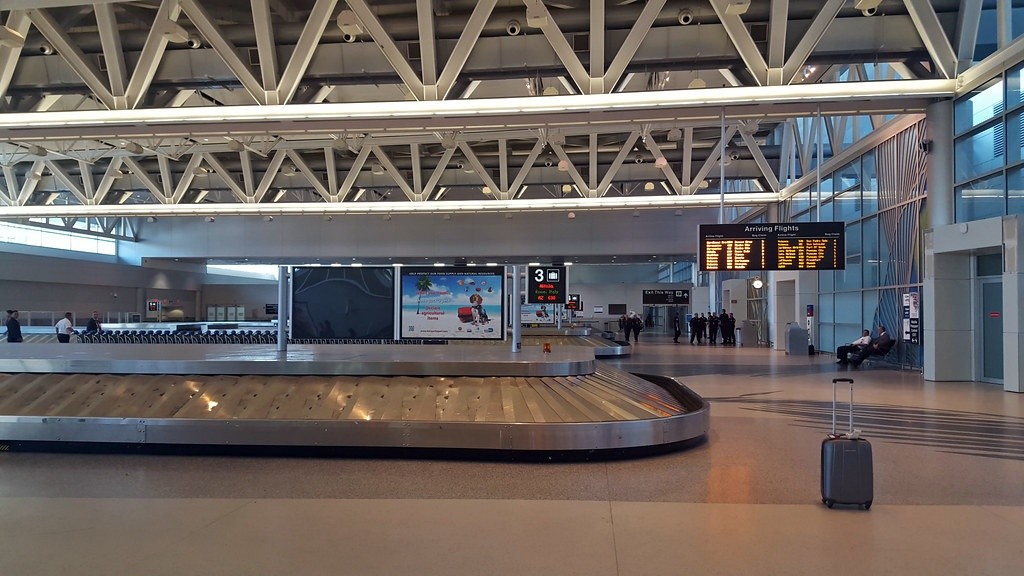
845;339;896;367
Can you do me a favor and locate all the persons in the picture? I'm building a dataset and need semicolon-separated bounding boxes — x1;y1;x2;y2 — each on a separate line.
53;312;74;344
86;311;100;330
618;313;654;342
848;326;890;367
672;312;680;344
835;329;871;364
689;309;736;346
4;310;22;342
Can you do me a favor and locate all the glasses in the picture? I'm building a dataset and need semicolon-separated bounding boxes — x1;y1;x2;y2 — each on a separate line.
14;313;18;316
94;314;98;316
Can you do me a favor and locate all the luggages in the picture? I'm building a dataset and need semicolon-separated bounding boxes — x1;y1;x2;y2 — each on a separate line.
820;378;873;510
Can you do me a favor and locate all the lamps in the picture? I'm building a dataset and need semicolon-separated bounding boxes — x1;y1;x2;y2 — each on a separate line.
723;0;751;14
25;145;731;223
526;0;547;28
0;24;25;48
543;79;559;96
160;0;188;43
854;0;882;9
667;118;681;141
687;22;706;89
337;4;363;35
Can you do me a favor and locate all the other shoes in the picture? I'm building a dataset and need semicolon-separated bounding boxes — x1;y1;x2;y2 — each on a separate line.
709;342;714;345
836;360;841;364
634;340;638;343
673;338;676;340
714;341;717;344
698;341;702;344
675;342;680;344
690;343;695;345
848;359;858;367
